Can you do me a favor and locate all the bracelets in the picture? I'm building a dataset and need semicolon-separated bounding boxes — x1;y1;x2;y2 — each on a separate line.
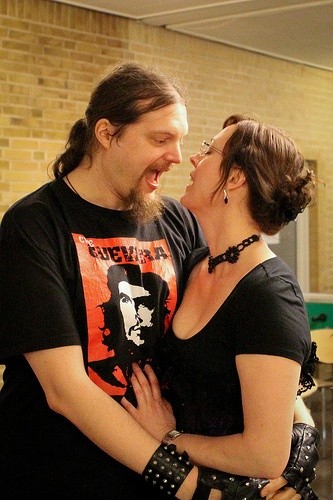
140;442;194;497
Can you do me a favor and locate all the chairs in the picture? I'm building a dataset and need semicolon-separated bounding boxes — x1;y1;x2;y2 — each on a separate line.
297;328;333;458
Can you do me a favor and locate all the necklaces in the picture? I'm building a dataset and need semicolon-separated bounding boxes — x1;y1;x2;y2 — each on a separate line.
66;173;80;197
207;233;261;275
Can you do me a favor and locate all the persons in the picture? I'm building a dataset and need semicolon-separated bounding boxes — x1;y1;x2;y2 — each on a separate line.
119;113;317;500
0;63;324;500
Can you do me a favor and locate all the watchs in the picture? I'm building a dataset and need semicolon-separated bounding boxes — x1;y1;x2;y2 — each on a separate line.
161;429;184;447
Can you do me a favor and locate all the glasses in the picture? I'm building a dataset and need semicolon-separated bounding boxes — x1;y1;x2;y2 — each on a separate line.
200;141;225;157
